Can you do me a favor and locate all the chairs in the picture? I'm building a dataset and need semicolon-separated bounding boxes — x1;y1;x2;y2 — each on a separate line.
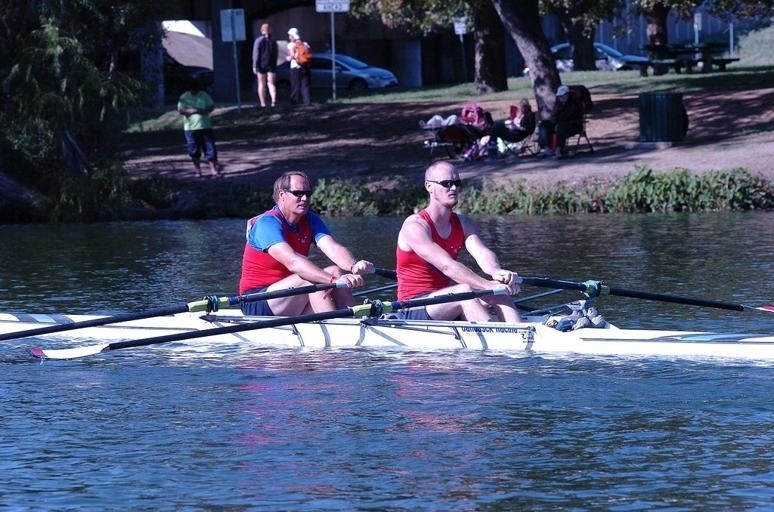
424;80;594;172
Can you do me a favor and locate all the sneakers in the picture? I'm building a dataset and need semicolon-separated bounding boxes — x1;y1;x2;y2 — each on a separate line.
538;149;563;160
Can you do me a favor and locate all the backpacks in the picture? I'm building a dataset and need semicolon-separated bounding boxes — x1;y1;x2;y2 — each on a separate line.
292;41;313;65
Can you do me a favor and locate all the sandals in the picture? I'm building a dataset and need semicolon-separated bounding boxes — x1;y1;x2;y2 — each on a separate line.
194;169;201;177
211;170;222;178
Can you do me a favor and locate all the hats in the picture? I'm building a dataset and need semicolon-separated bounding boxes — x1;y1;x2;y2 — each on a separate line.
555;85;569;96
287;27;301;39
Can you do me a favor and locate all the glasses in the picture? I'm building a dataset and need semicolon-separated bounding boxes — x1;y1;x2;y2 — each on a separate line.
428;179;461;188
288;190;313;197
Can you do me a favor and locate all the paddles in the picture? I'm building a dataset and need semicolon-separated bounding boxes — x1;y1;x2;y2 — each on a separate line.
29;284;508;359
517;277;773;313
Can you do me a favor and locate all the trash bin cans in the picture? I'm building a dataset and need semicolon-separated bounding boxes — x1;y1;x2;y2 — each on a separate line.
636;89;692;144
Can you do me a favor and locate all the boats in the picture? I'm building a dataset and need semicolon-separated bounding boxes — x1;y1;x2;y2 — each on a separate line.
1;307;774;369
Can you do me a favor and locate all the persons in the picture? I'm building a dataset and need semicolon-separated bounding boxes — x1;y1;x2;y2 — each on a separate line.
419;86;583;159
177;79;222;178
286;28;312;106
239;171;373;317
396;162;522;322
253;24;278;108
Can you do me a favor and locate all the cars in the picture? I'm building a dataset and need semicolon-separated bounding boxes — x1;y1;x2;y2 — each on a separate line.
251;52;398;97
523;41;647;75
